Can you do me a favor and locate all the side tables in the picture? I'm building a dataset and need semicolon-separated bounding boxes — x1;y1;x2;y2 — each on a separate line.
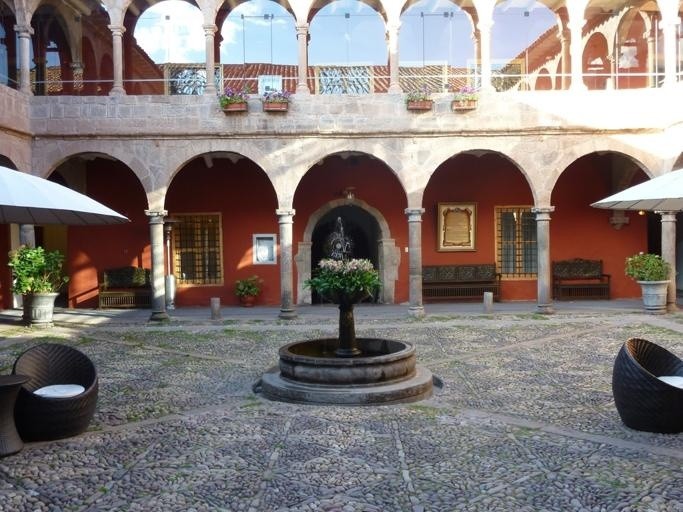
0;373;33;458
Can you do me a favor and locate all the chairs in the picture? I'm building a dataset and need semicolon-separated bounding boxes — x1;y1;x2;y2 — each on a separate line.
612;337;682;435
9;342;99;442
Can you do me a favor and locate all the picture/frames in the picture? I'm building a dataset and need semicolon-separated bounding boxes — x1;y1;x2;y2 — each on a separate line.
435;200;478;253
251;233;277;266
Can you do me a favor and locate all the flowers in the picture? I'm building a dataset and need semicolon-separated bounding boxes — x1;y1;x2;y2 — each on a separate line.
215;85;292;108
299;254;384;299
406;84;481;103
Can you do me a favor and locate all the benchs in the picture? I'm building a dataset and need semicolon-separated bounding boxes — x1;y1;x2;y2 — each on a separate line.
550;257;613;303
420;263;502;302
97;264;151;311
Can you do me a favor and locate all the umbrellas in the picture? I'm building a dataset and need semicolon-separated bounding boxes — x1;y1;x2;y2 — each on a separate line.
0;166;130;225
589;168;683;213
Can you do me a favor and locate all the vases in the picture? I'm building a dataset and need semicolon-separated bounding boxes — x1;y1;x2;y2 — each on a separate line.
449;101;476;111
222;103;287;113
406;101;433;110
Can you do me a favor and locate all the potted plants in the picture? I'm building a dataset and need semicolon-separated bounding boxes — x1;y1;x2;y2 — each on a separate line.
232;274;264;308
623;249;677;312
5;242;70;324
